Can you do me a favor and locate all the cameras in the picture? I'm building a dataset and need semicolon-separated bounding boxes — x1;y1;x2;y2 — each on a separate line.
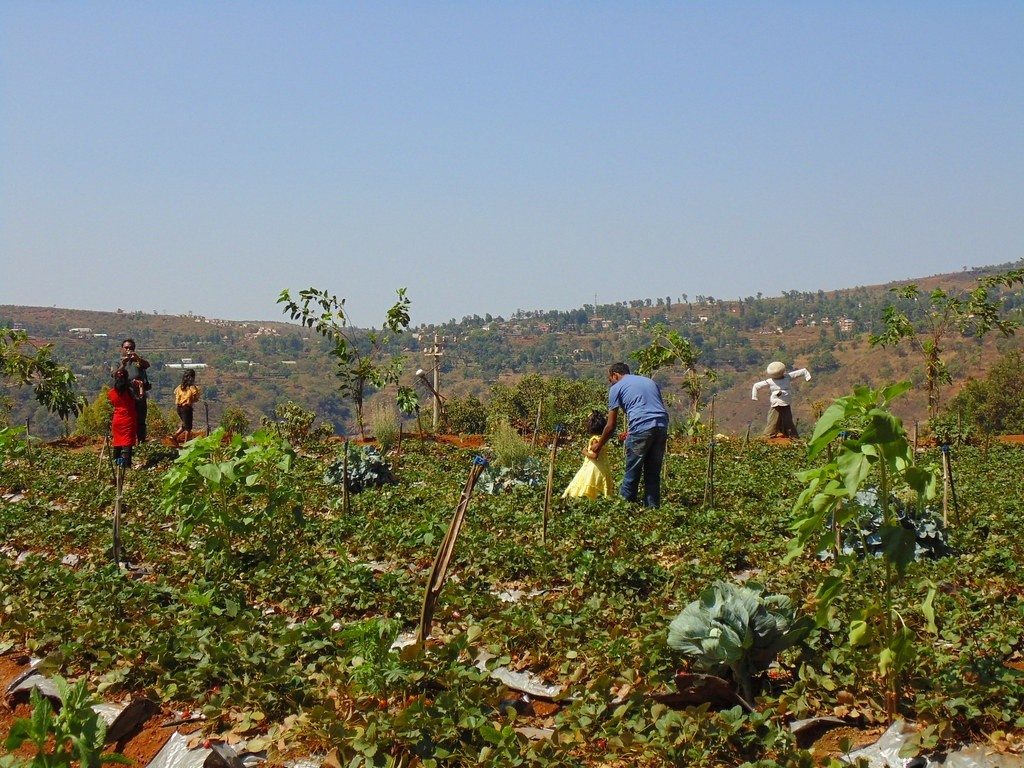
127;353;132;358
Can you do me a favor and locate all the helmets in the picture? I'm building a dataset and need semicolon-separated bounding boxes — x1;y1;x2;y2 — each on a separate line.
114;369;128;377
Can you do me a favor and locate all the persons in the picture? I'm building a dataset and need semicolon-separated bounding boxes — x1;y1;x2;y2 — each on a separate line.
110;340;150;444
751;362;811;438
108;369;144;469
590;362;669;509
169;369;200;446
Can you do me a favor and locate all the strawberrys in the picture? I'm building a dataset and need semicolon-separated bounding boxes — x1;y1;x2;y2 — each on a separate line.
597;739;605;748
163;686;270;749
378;690;432;710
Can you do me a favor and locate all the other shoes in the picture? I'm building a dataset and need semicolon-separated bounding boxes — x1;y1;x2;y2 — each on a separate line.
170;435;179;447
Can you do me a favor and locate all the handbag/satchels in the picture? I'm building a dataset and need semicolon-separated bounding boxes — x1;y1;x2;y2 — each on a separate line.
144;382;153;391
